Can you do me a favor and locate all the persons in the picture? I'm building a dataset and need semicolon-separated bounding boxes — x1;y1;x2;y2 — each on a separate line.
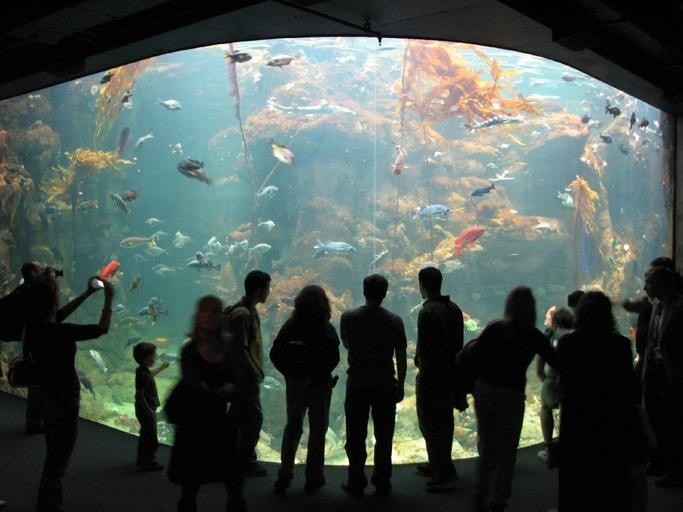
414;267;464;489
272;285;340;493
225;271;271;477
133;342;168;470
619;257;681;487
179;295;257;509
21;262;56;435
459;286;562;510
22;274;115;510
340;274;407;500
537;290;652;511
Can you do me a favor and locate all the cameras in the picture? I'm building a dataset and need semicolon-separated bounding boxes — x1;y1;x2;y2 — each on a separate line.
96;279;105;288
45;266;63;279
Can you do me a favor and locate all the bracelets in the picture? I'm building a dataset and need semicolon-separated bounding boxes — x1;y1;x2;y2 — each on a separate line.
101;307;112;313
